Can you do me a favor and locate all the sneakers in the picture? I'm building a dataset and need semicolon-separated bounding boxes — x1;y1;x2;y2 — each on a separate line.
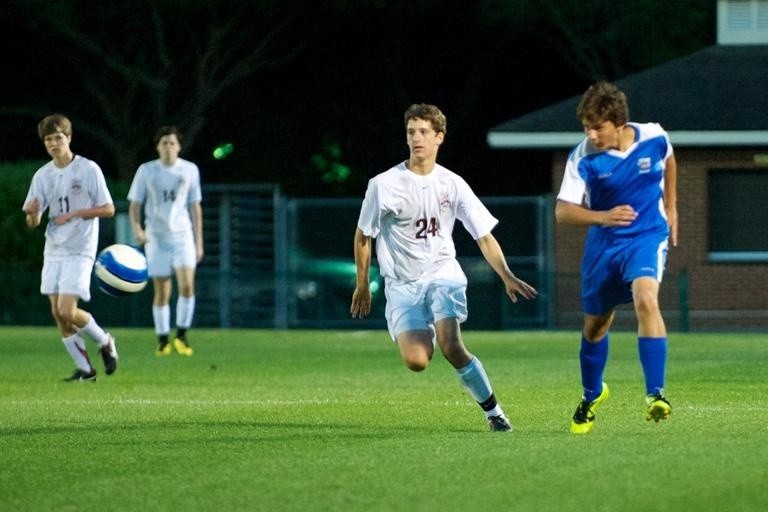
99;333;118;375
645;392;671;423
64;368;97;383
174;337;194;357
569;381;609;434
489;416;515;434
155;340;172;357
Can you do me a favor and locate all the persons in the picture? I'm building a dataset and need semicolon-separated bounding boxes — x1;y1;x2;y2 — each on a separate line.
126;123;208;359
350;104;540;434
21;114;119;386
554;82;681;434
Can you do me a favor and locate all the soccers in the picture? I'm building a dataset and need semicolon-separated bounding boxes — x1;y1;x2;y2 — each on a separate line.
93;243;148;297
